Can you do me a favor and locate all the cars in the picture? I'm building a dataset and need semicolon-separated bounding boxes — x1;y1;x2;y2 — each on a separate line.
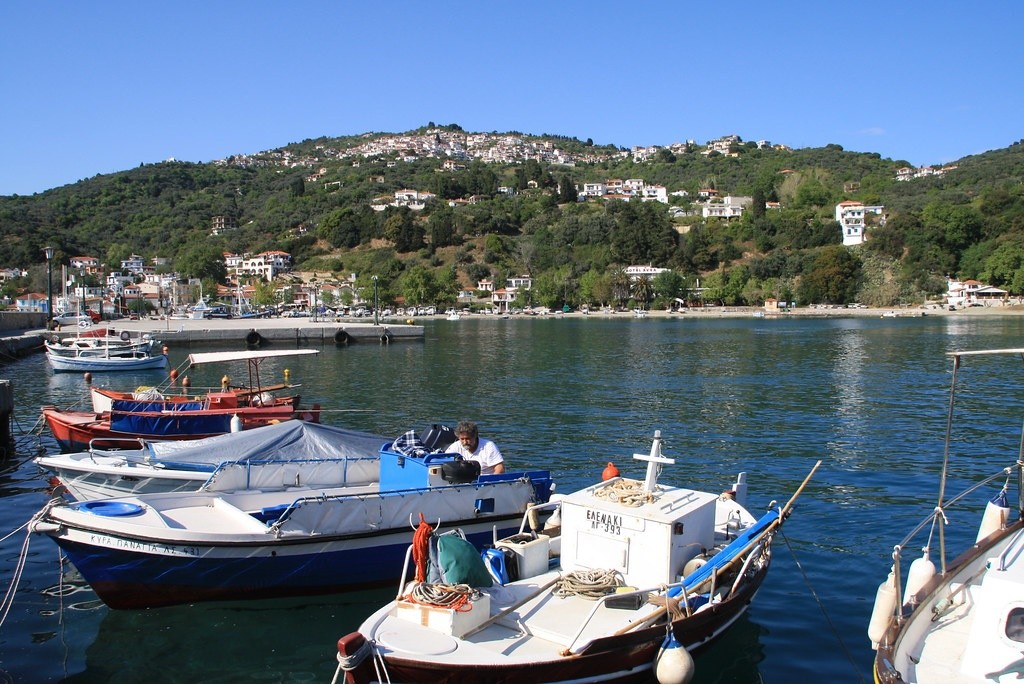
203;304;233;321
371;274;379;326
129;313;139;320
86;310;101;324
53;311;93;327
280;304;601;318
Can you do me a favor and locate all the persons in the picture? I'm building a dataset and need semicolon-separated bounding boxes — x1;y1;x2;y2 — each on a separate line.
445;422;505;474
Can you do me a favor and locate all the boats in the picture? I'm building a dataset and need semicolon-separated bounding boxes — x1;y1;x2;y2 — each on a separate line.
328;428;823;684
40;330;323;450
22;452;555;612
867;348;1024;684
19;418;455;502
232;267;273;318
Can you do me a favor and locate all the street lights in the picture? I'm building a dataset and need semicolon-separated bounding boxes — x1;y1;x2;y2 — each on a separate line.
136;287;141;320
78;268;88;311
44;246;55;330
